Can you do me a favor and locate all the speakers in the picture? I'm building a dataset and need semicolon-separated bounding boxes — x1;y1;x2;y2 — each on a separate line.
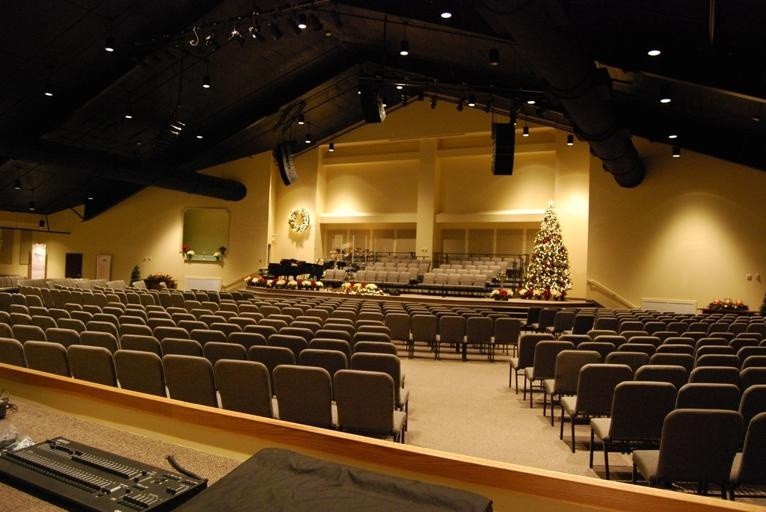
358;72;387;123
491;123;514;175
277;144;298;186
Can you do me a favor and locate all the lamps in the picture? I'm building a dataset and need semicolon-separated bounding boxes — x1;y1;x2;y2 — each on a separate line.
292;75;584;158
386;5;506;71
632;36;686;162
39;36;137;127
166;68;214;144
157;8;337;53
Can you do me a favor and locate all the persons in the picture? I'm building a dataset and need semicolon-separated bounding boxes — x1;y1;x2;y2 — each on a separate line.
213;246;226;256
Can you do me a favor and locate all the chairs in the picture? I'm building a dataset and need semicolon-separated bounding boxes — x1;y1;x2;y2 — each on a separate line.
509;301;765;503
412;299;509;365
268;253;531;300
2;274;413;443
